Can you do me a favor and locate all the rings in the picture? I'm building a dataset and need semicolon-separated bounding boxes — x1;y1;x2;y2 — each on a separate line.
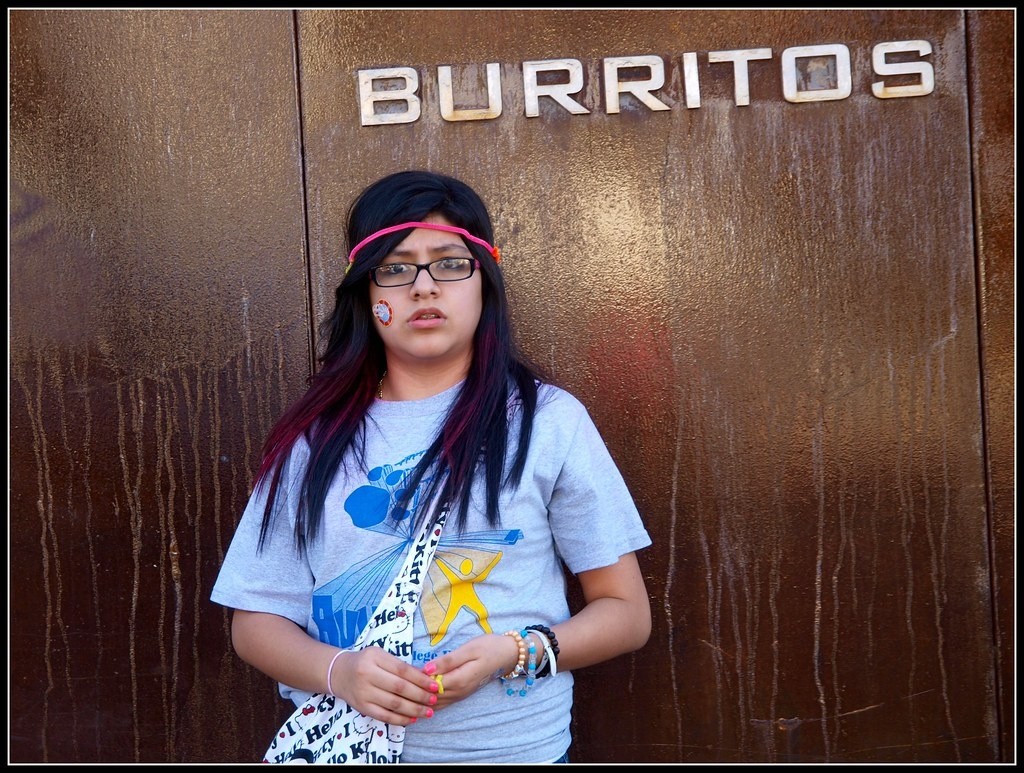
430;674;444;694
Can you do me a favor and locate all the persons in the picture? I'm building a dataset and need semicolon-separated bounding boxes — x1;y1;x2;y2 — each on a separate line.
210;171;655;763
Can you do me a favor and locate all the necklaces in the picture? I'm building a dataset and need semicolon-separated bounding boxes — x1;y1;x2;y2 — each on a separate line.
377;371;388;399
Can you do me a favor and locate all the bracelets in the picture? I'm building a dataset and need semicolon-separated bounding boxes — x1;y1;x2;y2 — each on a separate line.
498;624;560;697
327;649;351;695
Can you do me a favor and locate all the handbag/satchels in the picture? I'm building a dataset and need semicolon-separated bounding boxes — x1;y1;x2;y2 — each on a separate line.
262;628;414;765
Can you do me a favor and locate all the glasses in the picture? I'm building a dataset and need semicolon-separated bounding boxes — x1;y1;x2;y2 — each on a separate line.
368;257;480;287
